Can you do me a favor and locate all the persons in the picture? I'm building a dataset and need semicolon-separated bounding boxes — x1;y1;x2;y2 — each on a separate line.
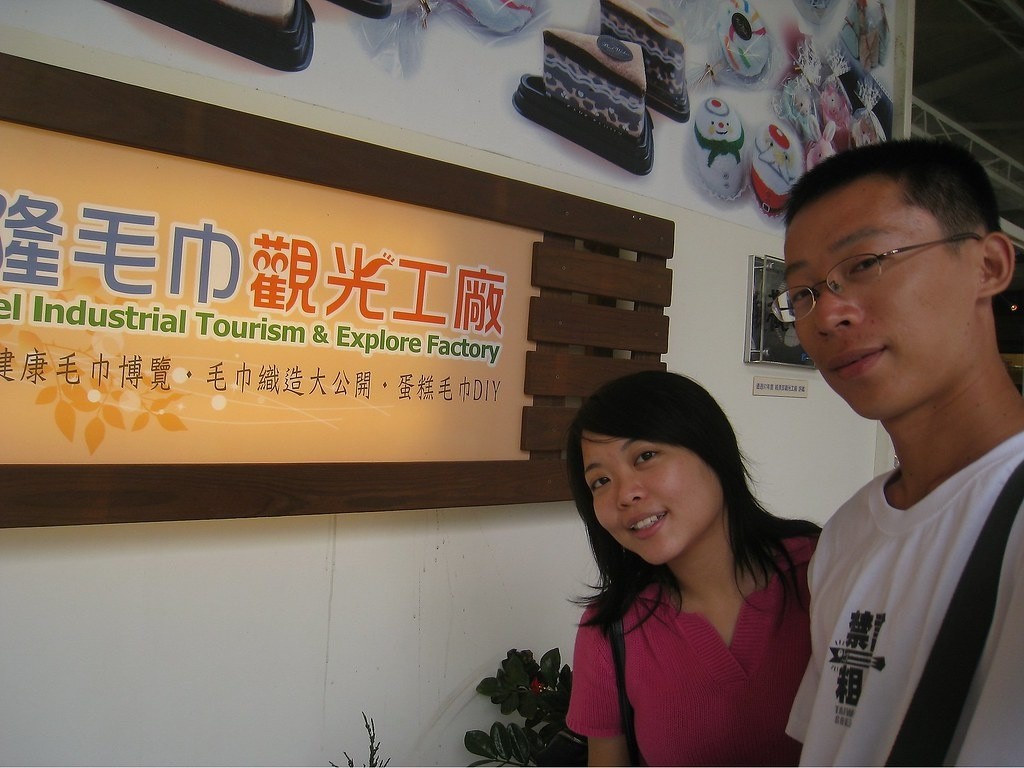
771;136;1024;767
566;371;822;768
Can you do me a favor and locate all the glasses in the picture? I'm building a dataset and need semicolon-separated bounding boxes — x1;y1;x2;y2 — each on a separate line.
770;231;983;323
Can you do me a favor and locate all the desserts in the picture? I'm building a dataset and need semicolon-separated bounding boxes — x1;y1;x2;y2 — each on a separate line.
216;0;890;212
